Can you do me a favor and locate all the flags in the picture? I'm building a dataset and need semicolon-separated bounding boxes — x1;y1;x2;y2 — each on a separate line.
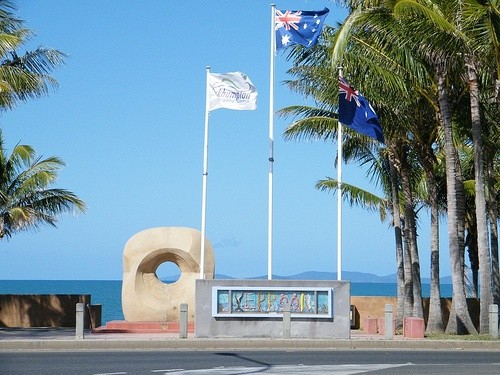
338;75;386;144
208;71;258;111
276;8;331;49
